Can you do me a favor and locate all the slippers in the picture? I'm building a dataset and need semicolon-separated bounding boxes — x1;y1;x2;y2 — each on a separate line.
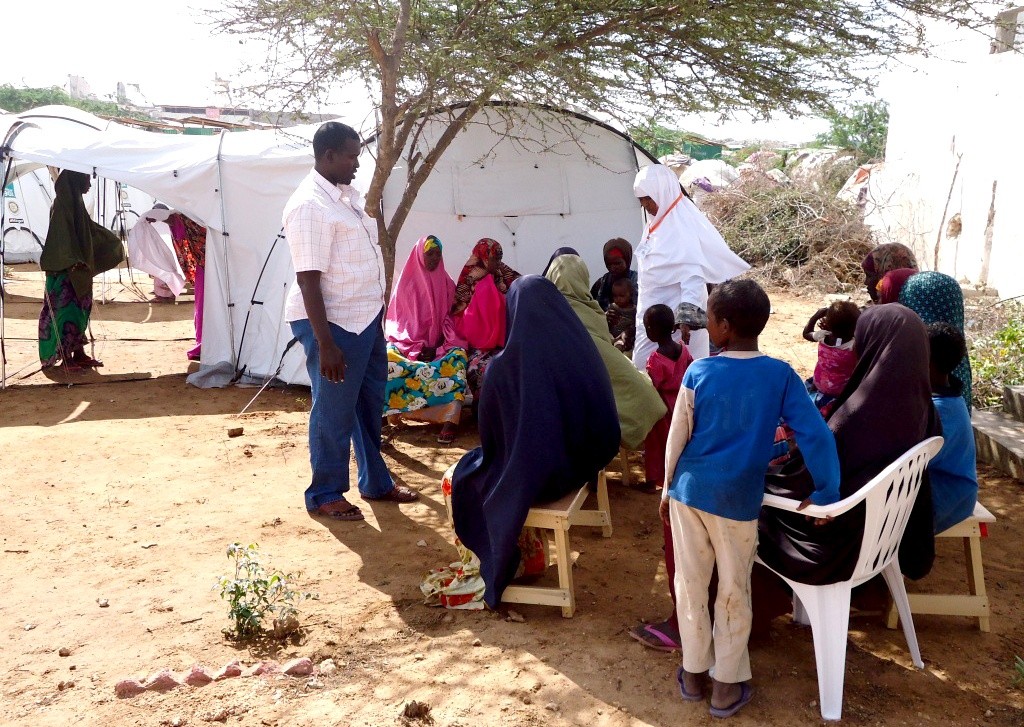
710;685;757;719
630;623;683;652
361;484;420;503
677;665;705;701
314;501;364;520
381;423;408;431
438;431;455;443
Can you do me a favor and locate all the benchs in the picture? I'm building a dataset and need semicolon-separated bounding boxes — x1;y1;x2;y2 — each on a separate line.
492;438;633;617
886;498;998;633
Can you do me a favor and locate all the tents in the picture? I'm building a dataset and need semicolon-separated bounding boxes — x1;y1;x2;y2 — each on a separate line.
836;52;1024;302
0;99;698;388
679;160;794;198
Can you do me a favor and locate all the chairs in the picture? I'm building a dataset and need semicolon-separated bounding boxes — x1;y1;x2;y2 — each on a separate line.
754;434;945;721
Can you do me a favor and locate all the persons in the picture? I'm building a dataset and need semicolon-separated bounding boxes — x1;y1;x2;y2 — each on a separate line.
629;243;978;720
382;164;751;493
37;170;123;372
146;204;206;361
280;121;421;519
441;274;621;584
